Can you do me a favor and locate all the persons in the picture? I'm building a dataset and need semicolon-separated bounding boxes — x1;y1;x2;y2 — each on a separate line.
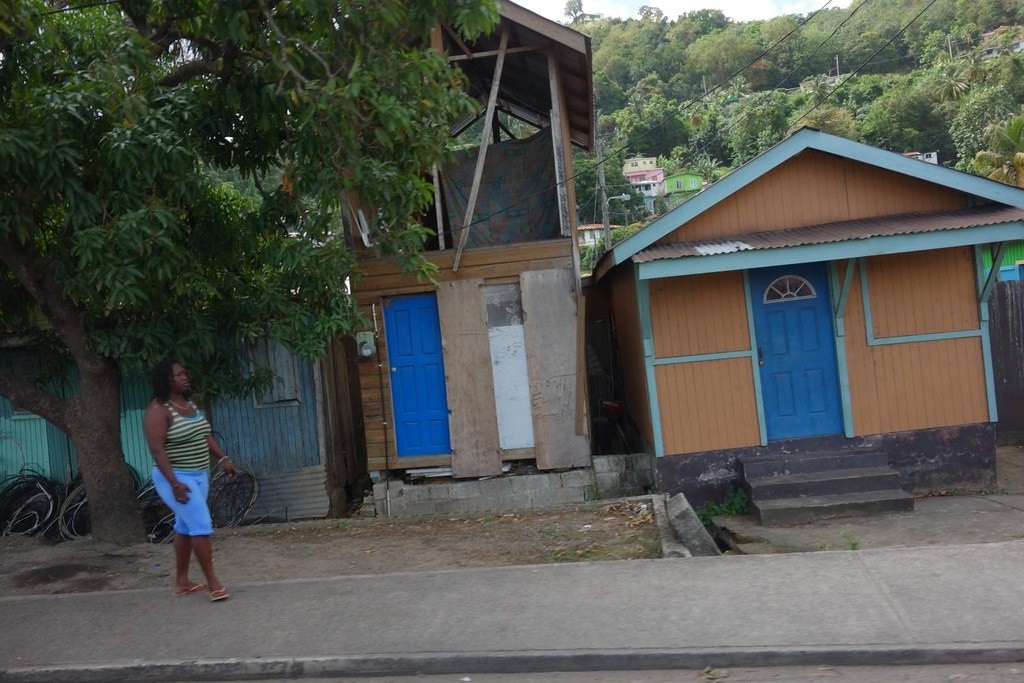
142;358;240;601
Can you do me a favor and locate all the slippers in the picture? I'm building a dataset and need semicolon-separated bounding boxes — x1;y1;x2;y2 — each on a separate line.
207;587;230;602
171;584;211;596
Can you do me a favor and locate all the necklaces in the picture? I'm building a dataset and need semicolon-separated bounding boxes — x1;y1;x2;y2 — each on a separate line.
169;400;191;410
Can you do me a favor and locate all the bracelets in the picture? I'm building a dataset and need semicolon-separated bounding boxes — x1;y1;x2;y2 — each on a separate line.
219;456;229;463
